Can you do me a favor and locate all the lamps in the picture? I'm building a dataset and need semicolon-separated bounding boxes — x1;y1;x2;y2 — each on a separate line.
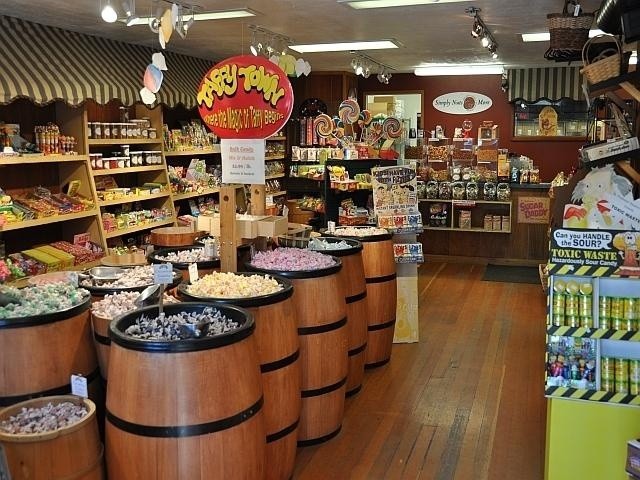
467;5;499;60
246;24;295;57
374;62;394;86
101;1;194;40
349;52;375;80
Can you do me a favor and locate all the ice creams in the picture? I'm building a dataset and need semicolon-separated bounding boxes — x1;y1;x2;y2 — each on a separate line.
339;100;359;142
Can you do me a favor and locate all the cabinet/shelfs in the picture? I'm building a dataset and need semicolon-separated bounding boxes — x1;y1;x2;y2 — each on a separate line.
324;157;398;228
0;101;285;291
286;142;416;215
580;0;640;188
415;111;513;234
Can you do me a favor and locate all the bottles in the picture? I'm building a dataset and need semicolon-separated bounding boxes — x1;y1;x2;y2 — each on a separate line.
142;151;152;166
152;151;157;164
127;123;133;139
122;123;127;139
141;120;148;139
102;158;110;169
142;116;151;128
92;122;101;139
88;122;92;139
130;119;141;139
110;151;122;157
117;157;125;168
124;157;130;168
147;128;157;139
110;123;122;139
102;123;110;139
96;153;104;169
157;151;162;164
129;151;137;167
120;145;129;157
133;123;137;139
138;151;142;166
110;158;118;169
89;153;96;169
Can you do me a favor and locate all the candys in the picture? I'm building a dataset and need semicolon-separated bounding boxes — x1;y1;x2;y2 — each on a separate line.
366;114;390;145
314;115;347;151
358;110;370;143
330;119;350;147
368;117;402;148
